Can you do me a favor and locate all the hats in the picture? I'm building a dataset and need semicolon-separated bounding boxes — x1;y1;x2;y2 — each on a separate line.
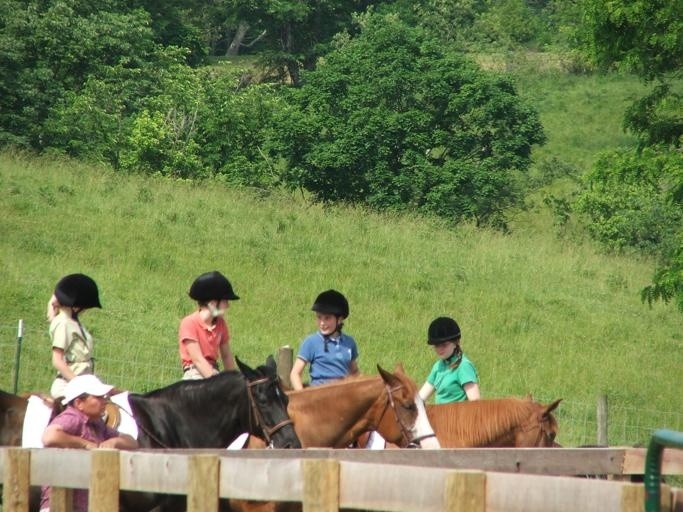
310;289;349;319
189;271;240;301
54;273;102;309
61;375;115;406
427;316;461;345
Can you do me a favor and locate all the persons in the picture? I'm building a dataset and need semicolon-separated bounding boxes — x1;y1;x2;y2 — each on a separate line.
46;273;101;401
39;375;140;512
178;270;240;381
289;290;361;391
417;317;481;405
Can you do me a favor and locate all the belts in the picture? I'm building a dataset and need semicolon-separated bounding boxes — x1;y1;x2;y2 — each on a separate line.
184;365;197;373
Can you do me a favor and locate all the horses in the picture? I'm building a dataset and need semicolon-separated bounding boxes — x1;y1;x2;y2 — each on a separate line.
0;355;301;512
227;362;443;512
346;393;564;450
